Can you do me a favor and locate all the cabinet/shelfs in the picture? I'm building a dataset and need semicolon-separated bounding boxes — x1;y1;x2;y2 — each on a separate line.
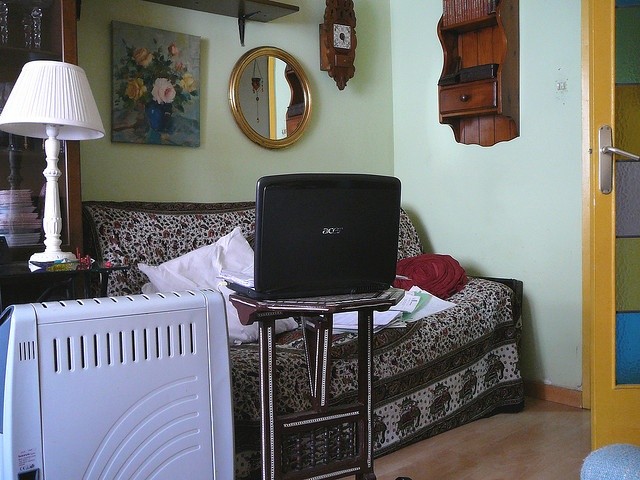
0;0;126;312
437;0;520;146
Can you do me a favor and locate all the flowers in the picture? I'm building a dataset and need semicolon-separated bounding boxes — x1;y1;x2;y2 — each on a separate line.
114;35;198;129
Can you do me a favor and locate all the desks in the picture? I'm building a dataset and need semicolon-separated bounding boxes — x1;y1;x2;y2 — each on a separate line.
229;287;404;478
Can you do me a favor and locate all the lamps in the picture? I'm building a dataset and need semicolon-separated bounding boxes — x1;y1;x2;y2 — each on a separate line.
3;58;106;272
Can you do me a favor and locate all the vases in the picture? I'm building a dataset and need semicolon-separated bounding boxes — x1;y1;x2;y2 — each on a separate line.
142;100;164;145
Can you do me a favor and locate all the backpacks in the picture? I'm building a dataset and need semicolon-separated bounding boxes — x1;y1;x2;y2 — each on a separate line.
393;254;467;299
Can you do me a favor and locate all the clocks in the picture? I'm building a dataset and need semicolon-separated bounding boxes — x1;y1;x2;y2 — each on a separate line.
318;0;358;90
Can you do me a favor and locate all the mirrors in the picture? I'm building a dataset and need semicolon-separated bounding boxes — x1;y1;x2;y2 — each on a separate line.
228;46;312;150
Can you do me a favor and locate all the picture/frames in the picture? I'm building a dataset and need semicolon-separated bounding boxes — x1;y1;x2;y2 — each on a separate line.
111;20;201;148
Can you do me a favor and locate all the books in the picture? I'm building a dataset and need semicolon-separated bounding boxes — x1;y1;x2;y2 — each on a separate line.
405;285;458;322
332;309;408;335
402;291;431;320
0;189;43;247
389;294;421;314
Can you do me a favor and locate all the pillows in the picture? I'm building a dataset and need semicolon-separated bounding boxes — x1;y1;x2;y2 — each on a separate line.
137;227;300;344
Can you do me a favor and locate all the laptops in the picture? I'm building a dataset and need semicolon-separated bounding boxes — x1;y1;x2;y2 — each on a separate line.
224;172;401;300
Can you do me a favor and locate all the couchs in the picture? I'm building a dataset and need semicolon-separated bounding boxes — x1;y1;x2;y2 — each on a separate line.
77;184;521;480
